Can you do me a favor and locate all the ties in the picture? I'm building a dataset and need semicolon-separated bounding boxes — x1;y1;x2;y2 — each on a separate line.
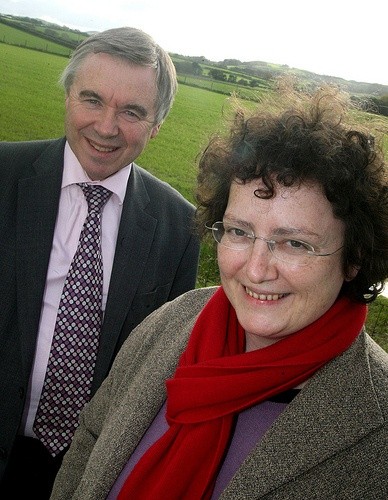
34;181;113;458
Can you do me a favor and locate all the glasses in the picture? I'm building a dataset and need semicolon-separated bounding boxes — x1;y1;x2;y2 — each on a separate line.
204;217;348;265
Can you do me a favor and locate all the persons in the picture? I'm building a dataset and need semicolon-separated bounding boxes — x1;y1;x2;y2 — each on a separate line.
0;26;201;500
49;73;388;500
